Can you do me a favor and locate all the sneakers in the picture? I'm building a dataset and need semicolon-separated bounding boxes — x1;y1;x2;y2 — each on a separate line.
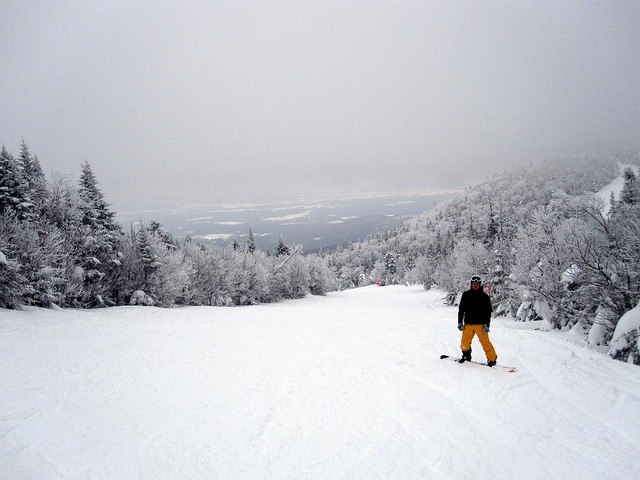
487;355;497;367
459;347;472;363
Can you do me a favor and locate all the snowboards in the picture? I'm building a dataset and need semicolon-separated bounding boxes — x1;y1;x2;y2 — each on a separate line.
440;354;516;372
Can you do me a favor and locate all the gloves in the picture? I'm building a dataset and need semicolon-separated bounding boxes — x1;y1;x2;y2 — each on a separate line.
458;323;464;331
483;324;490;333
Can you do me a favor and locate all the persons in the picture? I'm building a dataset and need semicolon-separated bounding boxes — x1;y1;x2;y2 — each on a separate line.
458;275;498;367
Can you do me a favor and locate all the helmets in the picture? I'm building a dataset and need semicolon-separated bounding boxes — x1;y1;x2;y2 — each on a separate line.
471;275;482;287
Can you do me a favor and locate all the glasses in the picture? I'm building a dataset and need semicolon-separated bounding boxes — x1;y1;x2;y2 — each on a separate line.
471;276;482;282
